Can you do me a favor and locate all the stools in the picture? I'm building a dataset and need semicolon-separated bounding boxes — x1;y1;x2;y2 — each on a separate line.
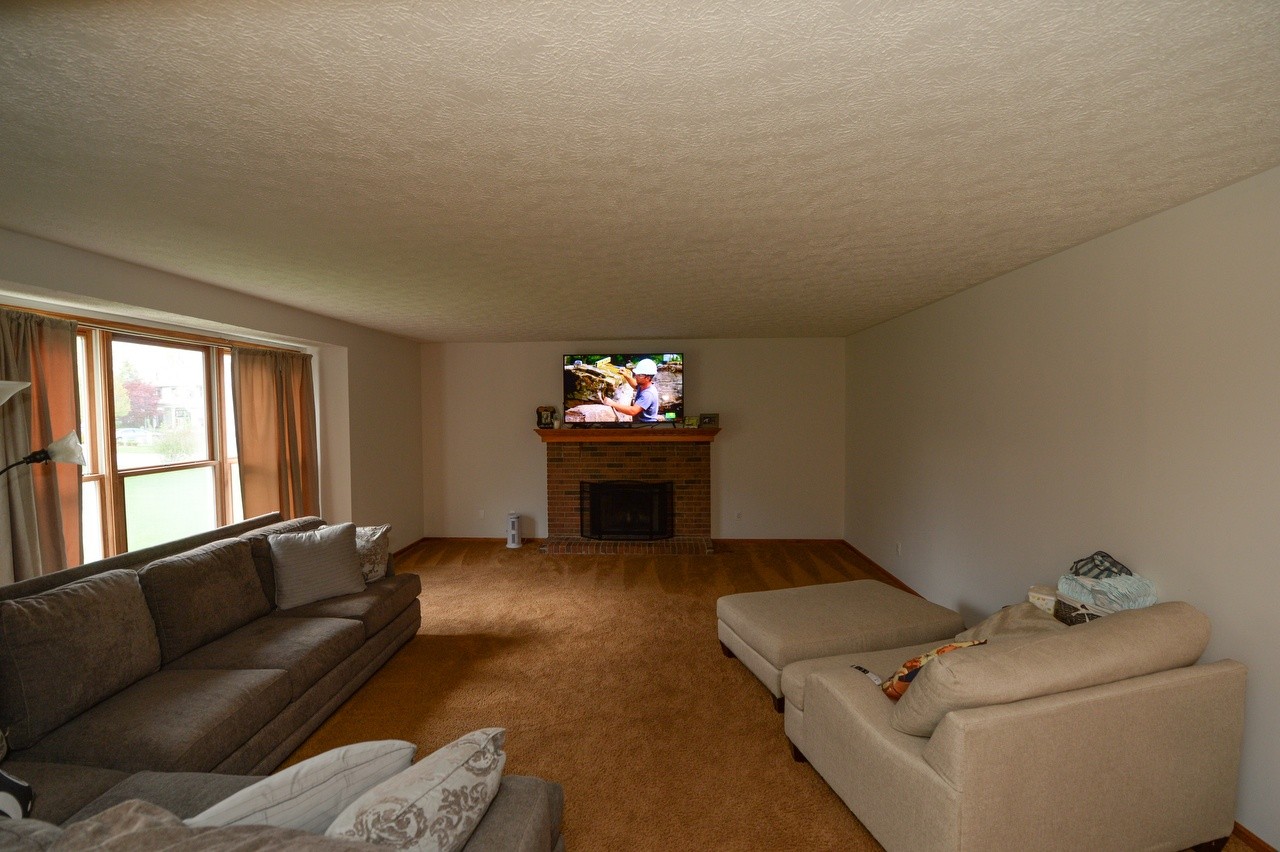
717;577;962;713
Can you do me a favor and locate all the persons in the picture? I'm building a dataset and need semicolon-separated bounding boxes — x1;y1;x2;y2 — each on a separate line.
602;359;659;422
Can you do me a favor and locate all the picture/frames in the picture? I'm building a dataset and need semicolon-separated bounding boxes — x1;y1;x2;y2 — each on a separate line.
536;405;555;429
699;412;720;429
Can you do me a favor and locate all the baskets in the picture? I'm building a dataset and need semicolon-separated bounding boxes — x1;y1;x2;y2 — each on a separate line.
1053;590;1115;627
1029;592;1056;615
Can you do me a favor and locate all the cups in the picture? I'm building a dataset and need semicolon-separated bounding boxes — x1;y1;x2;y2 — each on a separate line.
553;413;561;429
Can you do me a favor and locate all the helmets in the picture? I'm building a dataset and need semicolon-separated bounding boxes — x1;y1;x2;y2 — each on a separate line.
632;359;657;375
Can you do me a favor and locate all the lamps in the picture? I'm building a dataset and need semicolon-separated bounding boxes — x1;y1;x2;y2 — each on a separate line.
0;380;87;474
506;512;523;549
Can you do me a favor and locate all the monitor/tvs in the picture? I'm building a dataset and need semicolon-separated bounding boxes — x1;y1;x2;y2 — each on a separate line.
562;352;684;428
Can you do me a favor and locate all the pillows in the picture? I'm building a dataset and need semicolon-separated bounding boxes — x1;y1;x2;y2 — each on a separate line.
318;523;393;583
881;638;987;702
267;522;367;610
955;600;1075;643
182;738;418;831
322;728;505;852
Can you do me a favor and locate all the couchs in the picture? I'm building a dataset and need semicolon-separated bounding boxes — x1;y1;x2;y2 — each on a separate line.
780;598;1248;851
0;510;565;851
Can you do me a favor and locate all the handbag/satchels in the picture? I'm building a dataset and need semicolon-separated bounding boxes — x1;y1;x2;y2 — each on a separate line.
1069;551;1132;579
